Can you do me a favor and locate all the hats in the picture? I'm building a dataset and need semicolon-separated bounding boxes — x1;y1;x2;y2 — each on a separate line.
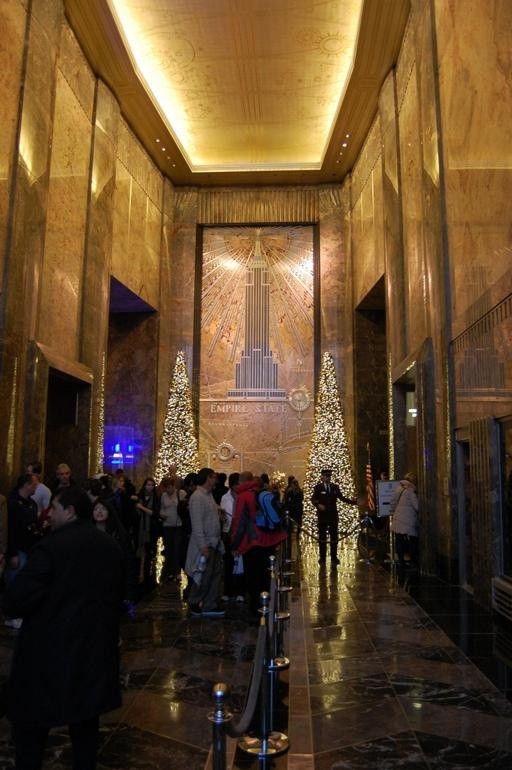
321;469;333;476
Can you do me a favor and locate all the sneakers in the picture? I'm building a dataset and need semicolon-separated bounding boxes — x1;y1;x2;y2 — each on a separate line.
186;594;244;617
3;617;23;630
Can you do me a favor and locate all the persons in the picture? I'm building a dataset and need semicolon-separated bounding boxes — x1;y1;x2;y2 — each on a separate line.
391;471;420;568
311;469;358;564
379;470;390;482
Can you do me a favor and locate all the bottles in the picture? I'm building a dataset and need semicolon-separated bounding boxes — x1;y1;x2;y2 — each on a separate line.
199;552;208;573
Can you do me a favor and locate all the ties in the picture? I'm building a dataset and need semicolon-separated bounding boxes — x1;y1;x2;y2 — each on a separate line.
326;485;329;493
